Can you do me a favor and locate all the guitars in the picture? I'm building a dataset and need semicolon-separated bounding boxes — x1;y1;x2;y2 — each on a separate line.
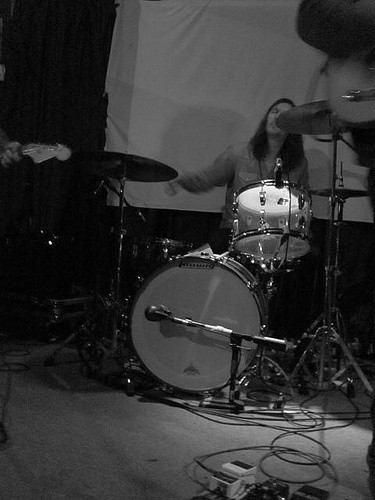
0;141;71;164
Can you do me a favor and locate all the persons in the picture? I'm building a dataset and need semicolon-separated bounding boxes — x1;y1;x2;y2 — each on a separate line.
0;129;24;170
164;97;326;368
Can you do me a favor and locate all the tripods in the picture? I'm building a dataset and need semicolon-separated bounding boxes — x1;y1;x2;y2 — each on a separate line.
277;128;375;401
43;163;129;369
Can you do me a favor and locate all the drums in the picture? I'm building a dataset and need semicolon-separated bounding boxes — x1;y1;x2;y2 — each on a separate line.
133;237;191;280
231;179;313;261
128;255;268;394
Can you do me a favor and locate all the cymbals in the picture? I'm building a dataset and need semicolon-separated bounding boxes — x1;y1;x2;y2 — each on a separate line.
82;150;176;183
310;188;370;199
275;95;356;134
